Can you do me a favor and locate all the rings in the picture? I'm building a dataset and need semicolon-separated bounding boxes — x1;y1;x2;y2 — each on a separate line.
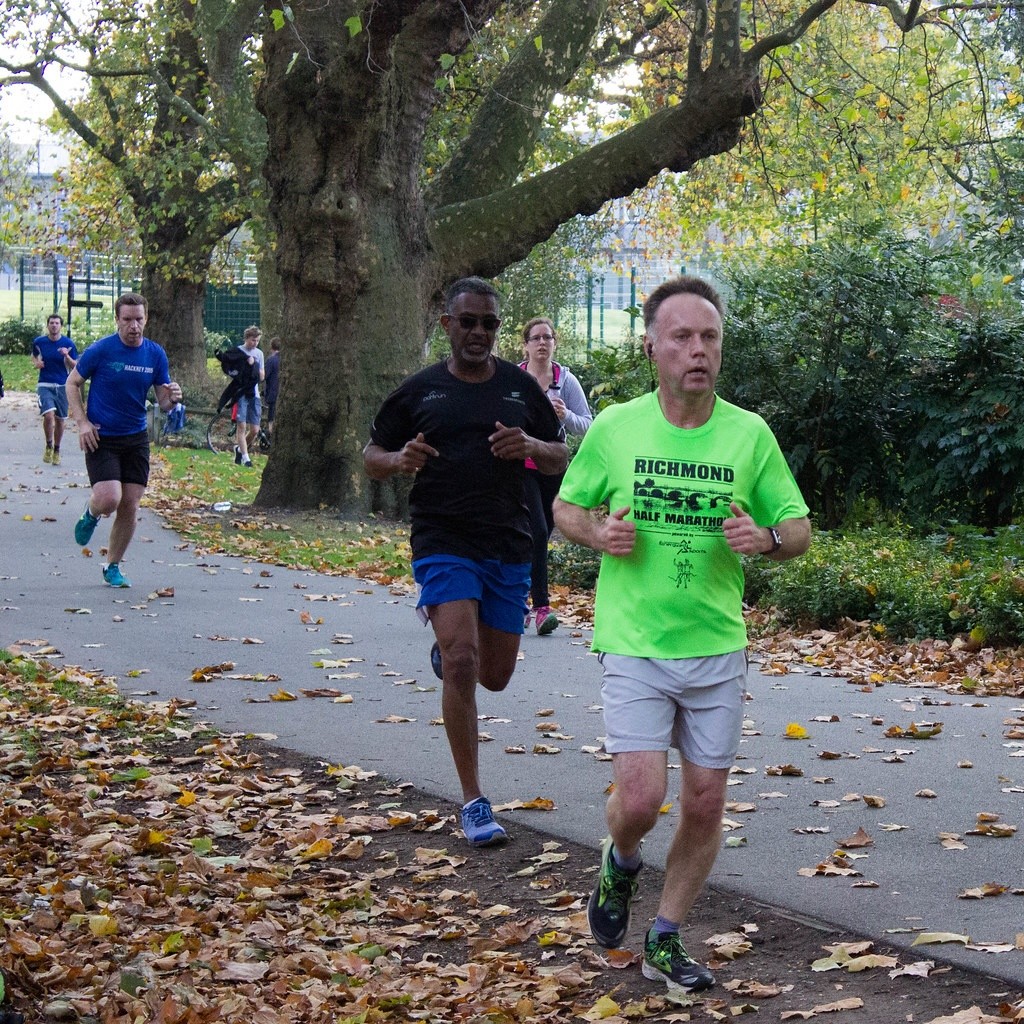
557;408;559;413
416;467;419;472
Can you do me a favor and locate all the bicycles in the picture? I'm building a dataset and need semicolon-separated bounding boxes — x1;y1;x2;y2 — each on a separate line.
207;391;272;455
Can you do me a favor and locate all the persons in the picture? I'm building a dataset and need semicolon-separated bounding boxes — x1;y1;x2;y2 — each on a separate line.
65;293;183;589
552;277;811;991
32;314;80;465
220;324;265;467
361;277;569;845
264;337;281;421
512;312;594;634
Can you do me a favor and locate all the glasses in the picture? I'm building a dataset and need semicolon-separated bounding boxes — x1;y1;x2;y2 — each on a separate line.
447;314;502;331
528;335;554;341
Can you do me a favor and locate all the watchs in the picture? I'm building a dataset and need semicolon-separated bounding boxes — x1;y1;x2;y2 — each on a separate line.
758;527;782;555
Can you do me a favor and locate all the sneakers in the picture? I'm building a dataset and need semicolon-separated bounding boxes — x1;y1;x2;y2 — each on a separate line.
52;451;61;465
103;561;132;588
641;928;717;992
43;446;54;463
533;606;559;635
431;641;443;680
586;834;645;949
74;507;101;545
460;797;510;848
241;459;251;467
233;445;242;464
524;601;531;627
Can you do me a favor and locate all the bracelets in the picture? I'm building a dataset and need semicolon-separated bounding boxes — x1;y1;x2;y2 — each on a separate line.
168;396;177;405
